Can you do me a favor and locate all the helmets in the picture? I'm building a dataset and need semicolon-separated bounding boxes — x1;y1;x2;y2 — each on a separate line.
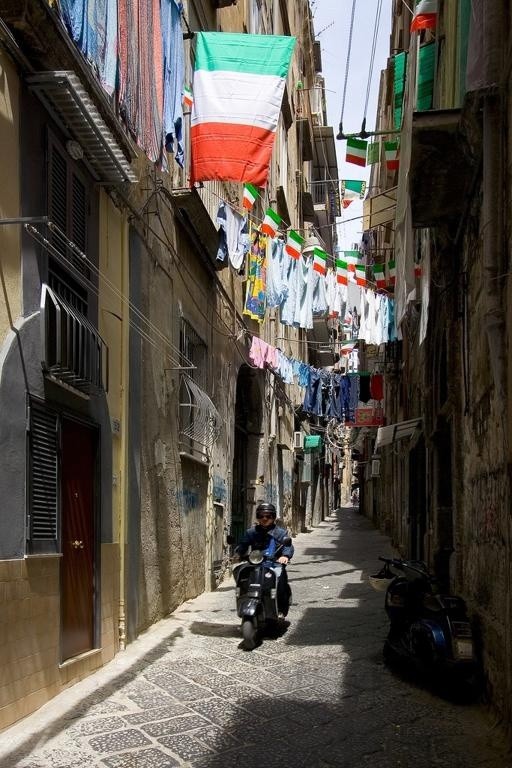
368;573;394;594
255;504;276;515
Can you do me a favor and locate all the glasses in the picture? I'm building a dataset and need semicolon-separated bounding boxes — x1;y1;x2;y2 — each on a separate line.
256;515;271;519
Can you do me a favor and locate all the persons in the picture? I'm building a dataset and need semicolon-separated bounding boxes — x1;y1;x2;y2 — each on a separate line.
232;503;294;621
352;494;358;508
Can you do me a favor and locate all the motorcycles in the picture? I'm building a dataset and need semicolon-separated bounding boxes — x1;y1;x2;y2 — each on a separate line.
376;555;488;706
225;533;294;649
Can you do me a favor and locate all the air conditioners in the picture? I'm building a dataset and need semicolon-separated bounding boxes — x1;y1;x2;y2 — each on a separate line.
294;432;304;451
372;460;380;475
